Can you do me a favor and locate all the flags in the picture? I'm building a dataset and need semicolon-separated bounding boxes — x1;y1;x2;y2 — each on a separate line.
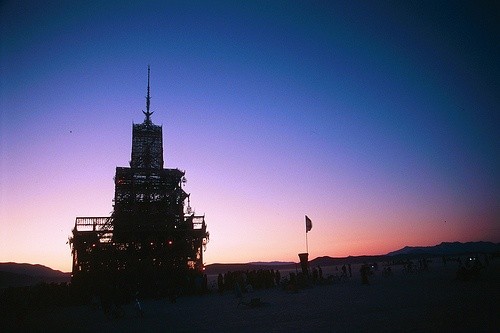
305;215;312;233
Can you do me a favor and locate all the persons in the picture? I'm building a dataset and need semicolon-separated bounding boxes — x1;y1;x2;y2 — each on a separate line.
204;251;499;291
134;292;145;319
